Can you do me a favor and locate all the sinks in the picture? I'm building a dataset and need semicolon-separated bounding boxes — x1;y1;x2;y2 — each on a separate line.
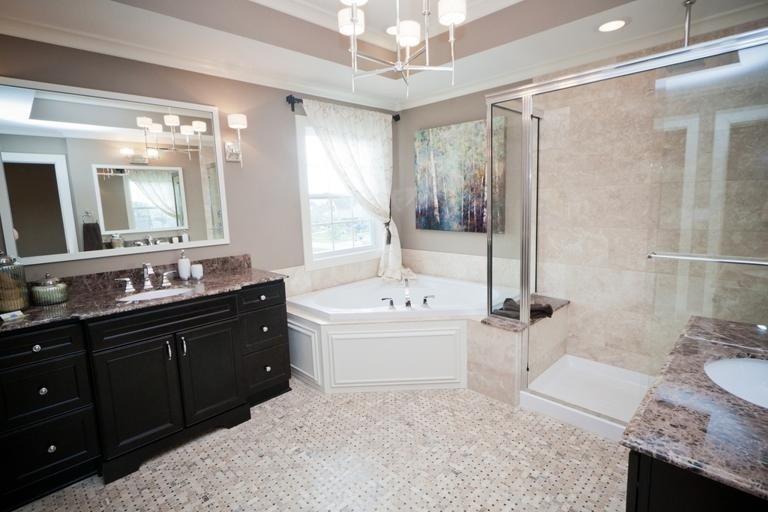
703;352;767;409
115;285;195;301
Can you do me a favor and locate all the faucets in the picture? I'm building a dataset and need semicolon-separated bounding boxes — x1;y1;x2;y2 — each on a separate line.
142;262;155;290
755;323;768;336
402;277;412;306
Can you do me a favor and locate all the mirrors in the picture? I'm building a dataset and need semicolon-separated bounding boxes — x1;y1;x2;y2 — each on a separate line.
0;76;231;267
91;164;190;236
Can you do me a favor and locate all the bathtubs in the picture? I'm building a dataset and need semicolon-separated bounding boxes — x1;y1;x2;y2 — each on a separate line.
286;272;519;319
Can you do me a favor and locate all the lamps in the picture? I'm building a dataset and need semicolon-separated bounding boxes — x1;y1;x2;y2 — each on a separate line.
136;114;207;160
338;0;467;97
224;113;247;168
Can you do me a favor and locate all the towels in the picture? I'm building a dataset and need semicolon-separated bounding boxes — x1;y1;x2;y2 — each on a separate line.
503;298;553;318
82;224;103;251
492;304;547;320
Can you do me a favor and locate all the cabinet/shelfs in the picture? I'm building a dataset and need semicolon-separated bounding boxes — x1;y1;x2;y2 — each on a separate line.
84;290;250;484
0;318;99;512
236;277;291;408
626;449;768;512
